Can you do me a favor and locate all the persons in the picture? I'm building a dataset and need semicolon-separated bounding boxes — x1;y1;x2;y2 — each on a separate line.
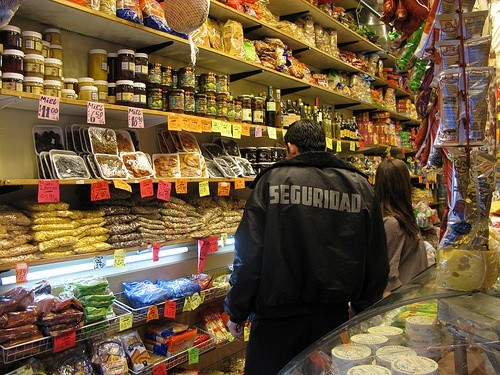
223;119;390;375
374;158;429;298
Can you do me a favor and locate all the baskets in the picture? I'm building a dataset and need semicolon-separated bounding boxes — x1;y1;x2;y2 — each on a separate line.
0;265;231;375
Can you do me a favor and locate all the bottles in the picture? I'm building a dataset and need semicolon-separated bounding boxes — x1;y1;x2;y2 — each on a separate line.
0;24;264;125
265;85;362;142
239;146;287;175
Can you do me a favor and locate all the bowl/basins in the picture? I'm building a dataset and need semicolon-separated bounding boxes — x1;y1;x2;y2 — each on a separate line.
30;123;257;181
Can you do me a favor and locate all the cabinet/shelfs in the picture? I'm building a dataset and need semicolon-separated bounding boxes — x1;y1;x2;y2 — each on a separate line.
0;0;424;375
